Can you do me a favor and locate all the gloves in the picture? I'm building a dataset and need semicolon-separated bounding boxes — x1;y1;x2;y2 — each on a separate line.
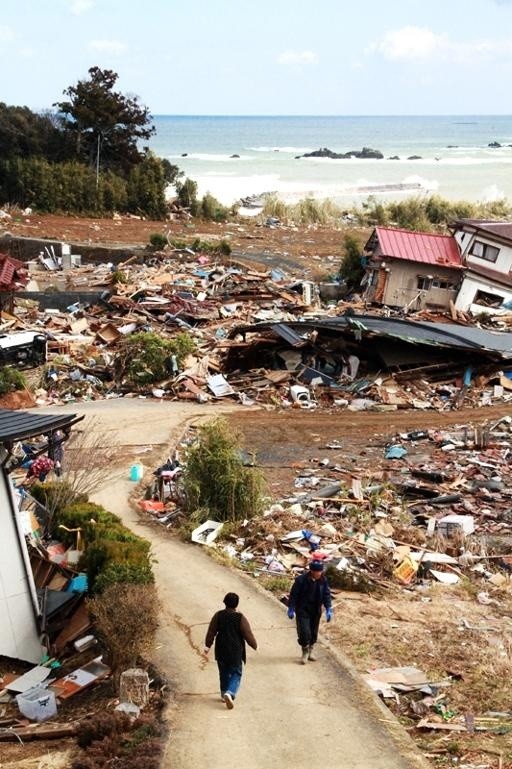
288;608;294;618
327;609;331;622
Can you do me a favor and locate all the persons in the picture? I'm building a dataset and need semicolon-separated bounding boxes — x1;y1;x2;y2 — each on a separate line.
204;592;258;709
288;560;333;664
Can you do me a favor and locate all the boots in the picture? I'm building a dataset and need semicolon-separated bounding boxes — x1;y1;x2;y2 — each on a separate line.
302;645;316;664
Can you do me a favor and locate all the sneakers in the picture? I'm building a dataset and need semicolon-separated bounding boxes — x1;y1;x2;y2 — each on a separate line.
224;693;233;709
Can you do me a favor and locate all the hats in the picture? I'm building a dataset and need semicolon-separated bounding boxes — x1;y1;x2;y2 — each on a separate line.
309;560;323;570
